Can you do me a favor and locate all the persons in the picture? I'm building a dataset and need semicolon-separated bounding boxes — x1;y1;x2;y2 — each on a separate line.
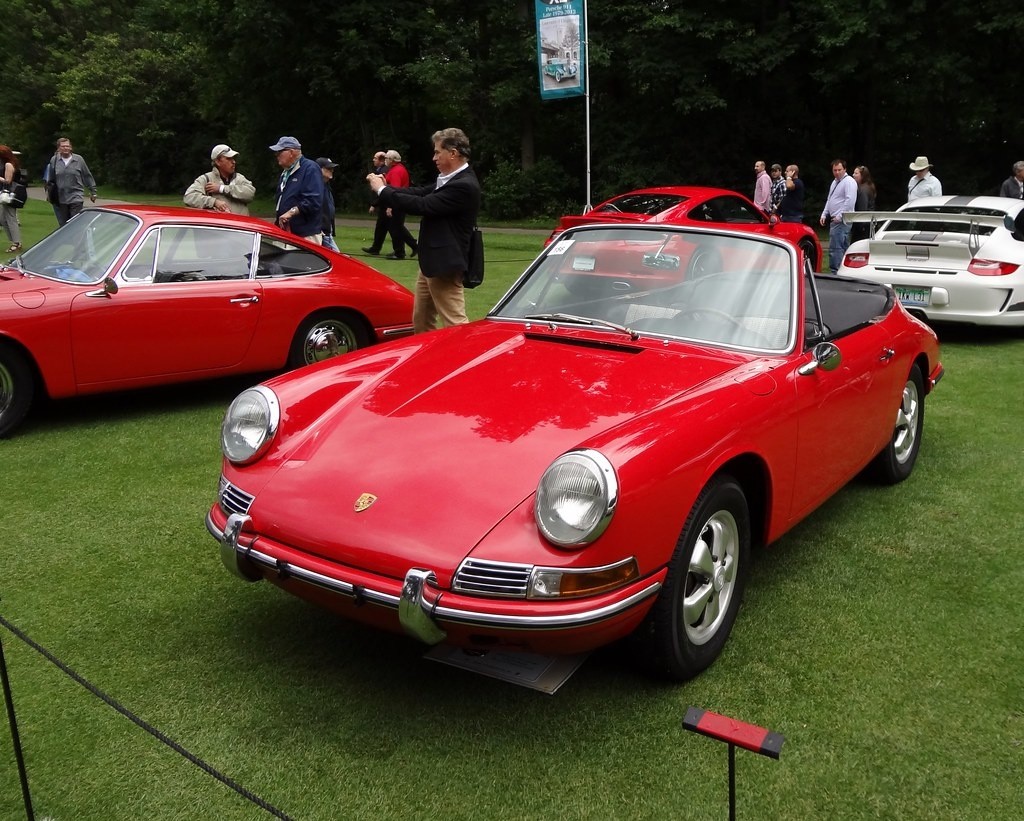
365;128;481;336
849;166;877;246
770;164;786;217
907;156;942;203
269;136;323;250
183;144;256;217
315;157;341;254
999;161;1024;201
0;144;27;253
753;161;774;218
776;165;805;223
819;159;858;275
362;149;419;260
44;137;98;228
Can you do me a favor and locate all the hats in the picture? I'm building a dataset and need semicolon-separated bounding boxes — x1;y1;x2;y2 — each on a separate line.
211;144;239;160
909;156;933;171
316;157;339;168
269;136;301;151
771;164;783;172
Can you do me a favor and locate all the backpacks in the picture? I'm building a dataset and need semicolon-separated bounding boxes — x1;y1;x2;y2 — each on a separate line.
8;181;27;208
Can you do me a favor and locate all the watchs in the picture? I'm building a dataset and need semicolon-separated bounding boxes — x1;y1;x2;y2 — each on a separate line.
290;207;298;214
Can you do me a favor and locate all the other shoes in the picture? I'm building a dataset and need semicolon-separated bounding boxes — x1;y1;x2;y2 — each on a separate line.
362;248;380;255
5;243;22;253
410;244;418;258
385;252;406;260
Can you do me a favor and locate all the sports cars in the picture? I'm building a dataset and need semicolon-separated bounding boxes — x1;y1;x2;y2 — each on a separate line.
837;195;1024;328
205;223;944;680
542;186;822;276
0;203;421;437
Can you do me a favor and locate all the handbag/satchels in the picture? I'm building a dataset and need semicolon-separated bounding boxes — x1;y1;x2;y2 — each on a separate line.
0;179;15;204
463;225;485;288
825;214;830;225
48;181;59;203
